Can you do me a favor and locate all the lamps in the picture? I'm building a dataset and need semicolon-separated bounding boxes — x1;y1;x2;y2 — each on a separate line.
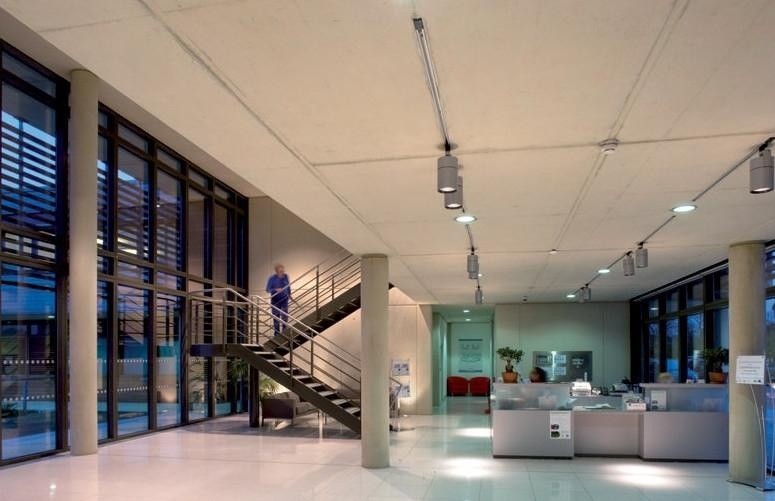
578;241;650;304
748;137;774;195
436;141;485;305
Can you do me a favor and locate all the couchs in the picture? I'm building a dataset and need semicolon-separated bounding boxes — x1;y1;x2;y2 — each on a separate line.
259;392;320;426
469;378;490;398
447;377;468;397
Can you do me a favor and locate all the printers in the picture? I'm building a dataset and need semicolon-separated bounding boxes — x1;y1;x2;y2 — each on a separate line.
572;381;592;397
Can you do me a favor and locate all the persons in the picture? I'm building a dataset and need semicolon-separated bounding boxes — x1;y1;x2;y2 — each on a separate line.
530;367;545;383
265;262;291;337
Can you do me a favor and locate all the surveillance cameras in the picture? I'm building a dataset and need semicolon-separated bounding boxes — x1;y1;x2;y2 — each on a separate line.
522;297;528;301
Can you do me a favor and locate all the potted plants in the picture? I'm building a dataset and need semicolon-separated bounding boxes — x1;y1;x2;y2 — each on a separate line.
495;346;529;383
701;345;729;384
